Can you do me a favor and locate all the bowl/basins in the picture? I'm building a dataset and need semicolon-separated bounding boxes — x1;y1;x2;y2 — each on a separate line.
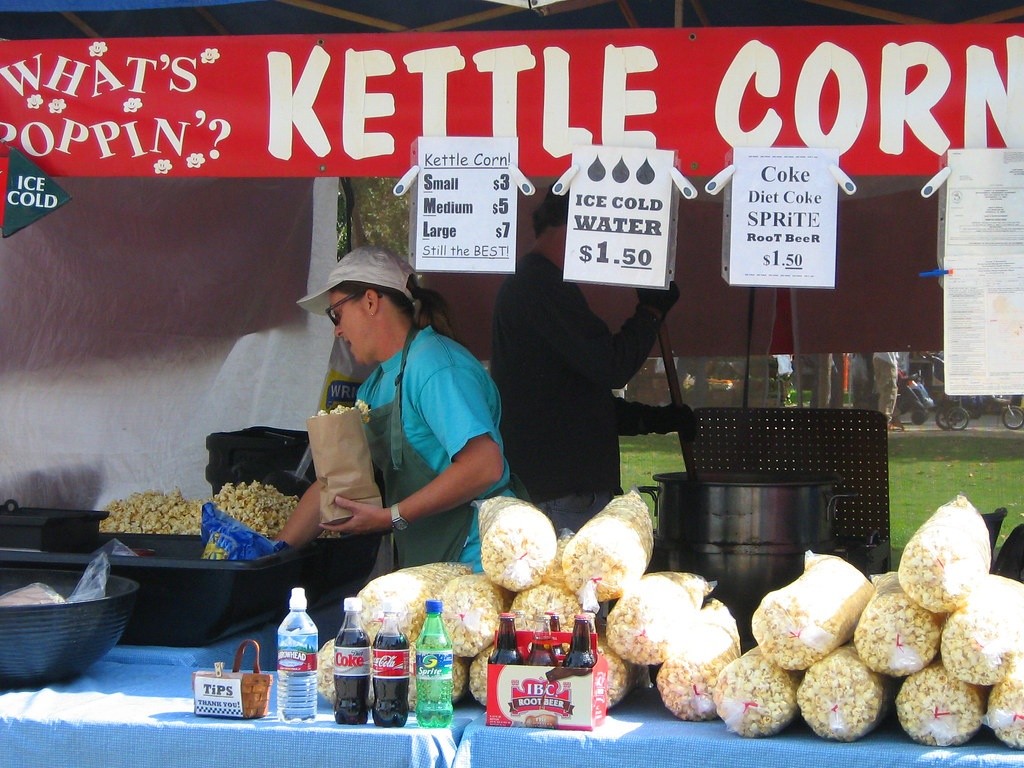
0;571;139;688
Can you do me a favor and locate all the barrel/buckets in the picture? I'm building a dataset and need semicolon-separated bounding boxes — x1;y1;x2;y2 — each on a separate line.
634;469;859;644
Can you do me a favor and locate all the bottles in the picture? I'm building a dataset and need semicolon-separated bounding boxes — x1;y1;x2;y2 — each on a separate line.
489;608;598;669
277;588;318;723
332;597;371;724
370;601;411;728
415;599;454;730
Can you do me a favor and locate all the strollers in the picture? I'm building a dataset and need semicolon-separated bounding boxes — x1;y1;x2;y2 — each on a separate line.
897;367;938;426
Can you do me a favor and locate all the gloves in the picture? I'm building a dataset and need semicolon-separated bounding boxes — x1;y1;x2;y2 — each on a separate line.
664;403;696;443
638;280;680;322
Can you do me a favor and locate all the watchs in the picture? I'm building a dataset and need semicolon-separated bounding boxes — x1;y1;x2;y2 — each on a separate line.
390;503;408;530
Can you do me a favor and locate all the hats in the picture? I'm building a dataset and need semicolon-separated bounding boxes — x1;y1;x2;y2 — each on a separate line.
296;244;418;315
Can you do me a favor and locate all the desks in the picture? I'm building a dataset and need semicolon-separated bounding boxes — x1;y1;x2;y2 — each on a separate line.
0;582;1024;768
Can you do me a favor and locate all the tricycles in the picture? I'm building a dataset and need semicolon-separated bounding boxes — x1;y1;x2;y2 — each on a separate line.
919;351;1024;432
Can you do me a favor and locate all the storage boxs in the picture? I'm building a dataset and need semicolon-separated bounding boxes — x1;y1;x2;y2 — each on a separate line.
0;532;385;649
0;500;110;548
485;631;609;730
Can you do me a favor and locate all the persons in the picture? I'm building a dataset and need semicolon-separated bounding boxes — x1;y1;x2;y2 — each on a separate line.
809;353;879;411
734;355;785;405
490;182;695;535
274;249;516;576
873;352;904;431
898;368;933;404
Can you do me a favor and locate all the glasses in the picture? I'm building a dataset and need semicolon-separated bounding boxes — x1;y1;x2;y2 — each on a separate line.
324;287;383;325
